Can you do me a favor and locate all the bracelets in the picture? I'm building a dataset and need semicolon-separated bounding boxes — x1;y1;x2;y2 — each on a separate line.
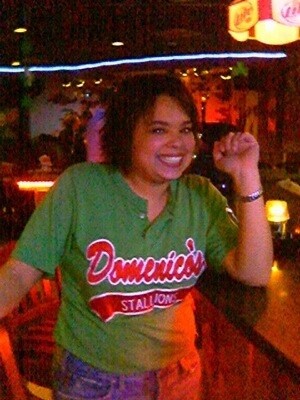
234;185;265;203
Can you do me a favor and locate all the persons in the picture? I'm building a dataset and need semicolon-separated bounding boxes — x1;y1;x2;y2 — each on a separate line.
1;67;274;399
0;87;117;173
